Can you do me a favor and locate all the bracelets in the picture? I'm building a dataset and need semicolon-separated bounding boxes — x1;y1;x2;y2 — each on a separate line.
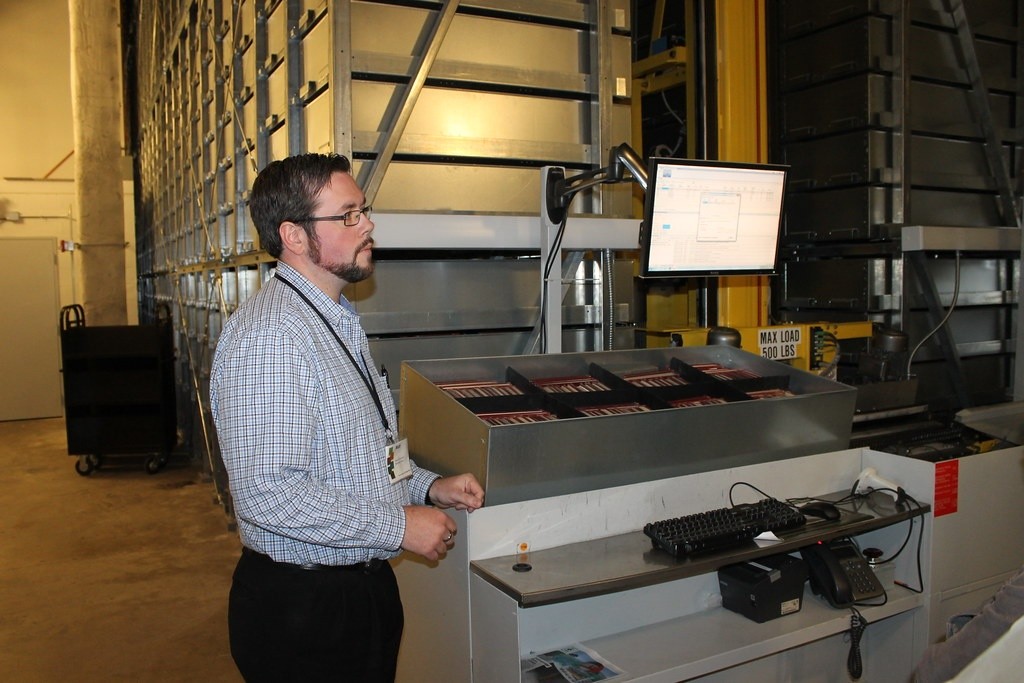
425;477;442;505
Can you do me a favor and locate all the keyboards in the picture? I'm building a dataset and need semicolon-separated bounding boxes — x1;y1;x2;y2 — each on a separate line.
644;498;806;559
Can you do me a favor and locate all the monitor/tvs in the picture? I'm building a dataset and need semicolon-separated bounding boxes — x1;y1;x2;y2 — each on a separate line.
638;157;792;279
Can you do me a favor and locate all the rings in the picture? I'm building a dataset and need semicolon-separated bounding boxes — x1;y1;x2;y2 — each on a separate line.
444;532;452;542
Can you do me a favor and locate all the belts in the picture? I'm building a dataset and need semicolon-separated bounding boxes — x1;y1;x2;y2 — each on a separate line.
282;559;381;572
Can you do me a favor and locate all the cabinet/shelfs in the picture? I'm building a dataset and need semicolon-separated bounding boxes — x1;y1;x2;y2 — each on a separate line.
470;487;932;683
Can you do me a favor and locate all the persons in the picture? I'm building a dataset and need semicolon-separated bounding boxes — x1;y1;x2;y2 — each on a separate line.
208;153;484;683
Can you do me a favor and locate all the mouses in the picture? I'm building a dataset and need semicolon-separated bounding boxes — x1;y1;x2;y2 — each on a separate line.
798;502;841;521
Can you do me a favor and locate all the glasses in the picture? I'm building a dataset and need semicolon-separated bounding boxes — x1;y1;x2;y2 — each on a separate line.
292;205;373;227
